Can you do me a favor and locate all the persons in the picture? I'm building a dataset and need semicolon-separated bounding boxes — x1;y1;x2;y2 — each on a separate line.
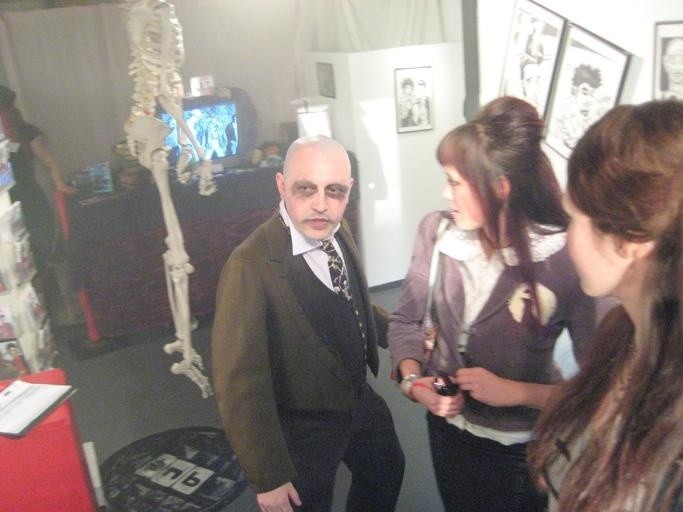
500;10;548;109
558;63;602;150
413;80;431;127
523;97;682;511
163;104;240;167
662;38;683;97
2;85;83;349
211;133;408;512
383;96;599;512
401;78;419;126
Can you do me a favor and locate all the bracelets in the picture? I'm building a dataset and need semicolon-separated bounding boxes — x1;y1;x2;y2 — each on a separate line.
400;375;423;403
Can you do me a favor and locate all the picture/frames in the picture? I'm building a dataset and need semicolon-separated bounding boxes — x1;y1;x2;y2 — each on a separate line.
496;0;683;161
393;65;437;134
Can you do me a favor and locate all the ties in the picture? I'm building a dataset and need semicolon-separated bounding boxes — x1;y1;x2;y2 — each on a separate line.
319;240;353;301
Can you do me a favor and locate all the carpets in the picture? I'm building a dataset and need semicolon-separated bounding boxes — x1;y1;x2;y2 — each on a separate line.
100;425;248;512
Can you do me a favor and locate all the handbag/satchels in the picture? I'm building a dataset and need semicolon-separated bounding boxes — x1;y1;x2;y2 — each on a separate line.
390;315;440;380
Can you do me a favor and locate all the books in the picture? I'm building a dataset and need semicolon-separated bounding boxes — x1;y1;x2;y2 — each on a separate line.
113;139;150;194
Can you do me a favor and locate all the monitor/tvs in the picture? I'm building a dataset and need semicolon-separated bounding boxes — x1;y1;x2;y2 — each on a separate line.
156;86;253;175
